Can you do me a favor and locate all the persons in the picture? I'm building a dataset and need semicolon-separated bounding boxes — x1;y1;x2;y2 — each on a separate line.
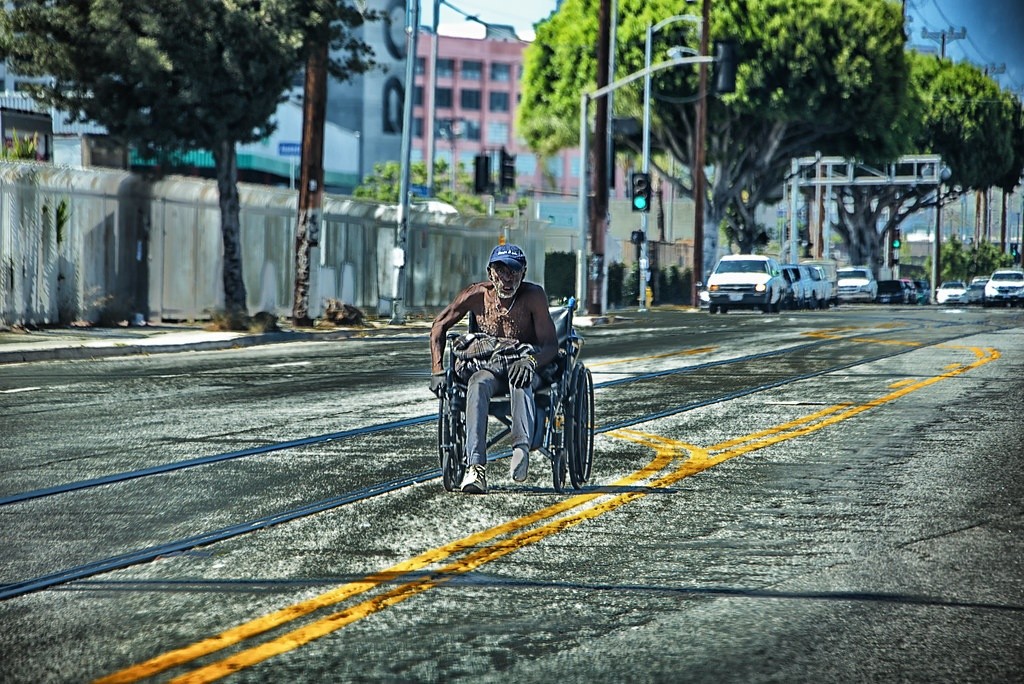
429;244;559;492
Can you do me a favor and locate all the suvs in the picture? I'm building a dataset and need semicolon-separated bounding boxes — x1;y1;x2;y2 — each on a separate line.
707;254;782;313
985;271;1024;308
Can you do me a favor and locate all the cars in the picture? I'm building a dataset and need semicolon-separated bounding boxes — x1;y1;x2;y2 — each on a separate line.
780;259;989;308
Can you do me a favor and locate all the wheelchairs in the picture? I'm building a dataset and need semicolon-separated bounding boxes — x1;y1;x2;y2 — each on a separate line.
436;296;594;493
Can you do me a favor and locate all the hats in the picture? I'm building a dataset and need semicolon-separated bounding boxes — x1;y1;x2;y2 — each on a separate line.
487;243;527;271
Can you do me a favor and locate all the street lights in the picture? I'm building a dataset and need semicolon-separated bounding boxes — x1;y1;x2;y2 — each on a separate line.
920;162;952;304
572;53;714;318
636;14;705;312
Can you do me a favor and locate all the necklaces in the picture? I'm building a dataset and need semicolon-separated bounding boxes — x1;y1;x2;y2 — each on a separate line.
495;292;517;315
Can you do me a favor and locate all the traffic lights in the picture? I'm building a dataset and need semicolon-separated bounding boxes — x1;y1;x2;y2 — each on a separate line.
1010;244;1018;257
500;147;515;193
892;229;901;249
632;173;650;212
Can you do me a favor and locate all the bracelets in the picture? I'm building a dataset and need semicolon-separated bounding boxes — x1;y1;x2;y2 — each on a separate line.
527;355;538;370
430;370;447;376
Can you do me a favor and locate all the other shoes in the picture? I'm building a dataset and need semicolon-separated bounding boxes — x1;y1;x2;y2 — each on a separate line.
460;462;487;493
510;447;529;481
